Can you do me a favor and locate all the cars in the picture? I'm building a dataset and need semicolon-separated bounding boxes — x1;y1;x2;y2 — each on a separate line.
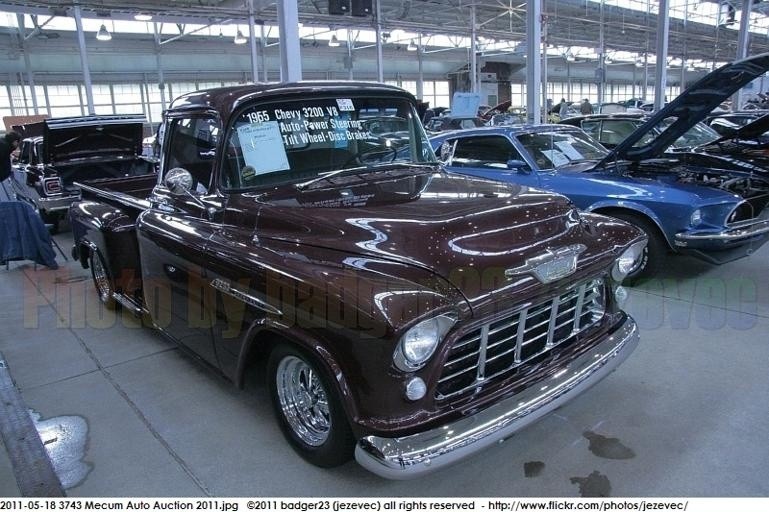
357;116;434;161
5;114;160;234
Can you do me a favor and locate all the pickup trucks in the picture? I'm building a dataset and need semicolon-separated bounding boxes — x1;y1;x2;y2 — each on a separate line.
68;80;640;482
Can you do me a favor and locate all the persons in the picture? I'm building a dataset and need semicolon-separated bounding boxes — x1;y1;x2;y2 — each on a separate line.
559;98;568;120
580;98;594;115
0;131;23;182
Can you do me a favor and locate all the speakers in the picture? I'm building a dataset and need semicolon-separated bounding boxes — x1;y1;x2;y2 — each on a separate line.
351;0;372;17
328;0;350;15
